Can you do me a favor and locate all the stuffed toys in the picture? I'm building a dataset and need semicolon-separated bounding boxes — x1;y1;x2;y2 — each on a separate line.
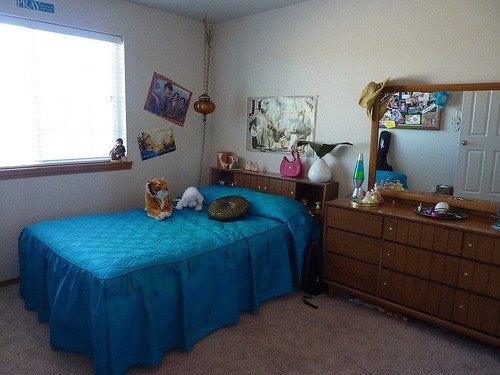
144;177;174;221
175;186;204;211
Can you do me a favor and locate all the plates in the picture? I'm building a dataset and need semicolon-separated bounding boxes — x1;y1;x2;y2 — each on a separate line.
350;198;384;207
413;206;468;220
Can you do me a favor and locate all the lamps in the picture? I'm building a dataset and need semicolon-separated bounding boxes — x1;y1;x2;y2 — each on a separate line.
193;13;215;188
348;152;366;199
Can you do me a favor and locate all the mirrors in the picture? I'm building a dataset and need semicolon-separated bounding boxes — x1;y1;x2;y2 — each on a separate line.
367;82;500;213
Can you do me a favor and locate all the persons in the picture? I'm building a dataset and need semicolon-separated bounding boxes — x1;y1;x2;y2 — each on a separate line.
109;138;125;160
152;82;186;120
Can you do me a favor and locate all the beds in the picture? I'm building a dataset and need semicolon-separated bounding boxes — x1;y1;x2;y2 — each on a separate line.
17;165;338;375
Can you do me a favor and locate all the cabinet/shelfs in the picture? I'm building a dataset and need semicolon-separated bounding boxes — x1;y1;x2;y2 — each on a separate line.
321;194;500;348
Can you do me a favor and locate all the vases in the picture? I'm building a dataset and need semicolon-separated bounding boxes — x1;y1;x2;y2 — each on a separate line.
308;156;332;184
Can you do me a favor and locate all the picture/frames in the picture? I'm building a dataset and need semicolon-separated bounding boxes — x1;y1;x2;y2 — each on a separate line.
379;91;441;130
144;72;193;127
